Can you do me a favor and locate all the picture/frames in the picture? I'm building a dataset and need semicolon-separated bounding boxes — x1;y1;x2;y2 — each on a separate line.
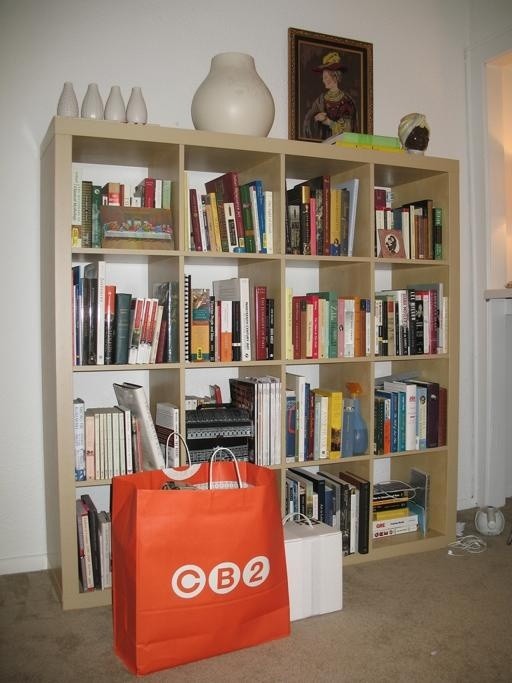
376;228;408;258
286;24;375;145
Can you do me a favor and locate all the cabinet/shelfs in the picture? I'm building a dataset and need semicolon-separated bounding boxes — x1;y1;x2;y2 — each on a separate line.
368;145;457;560
181;126;283;470
41;119;183;609
282;138;370;566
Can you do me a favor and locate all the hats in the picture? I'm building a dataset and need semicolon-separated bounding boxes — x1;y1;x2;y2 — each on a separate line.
312;50;347;73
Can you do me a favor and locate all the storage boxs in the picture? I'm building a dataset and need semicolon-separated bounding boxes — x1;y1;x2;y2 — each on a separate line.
280;512;344;622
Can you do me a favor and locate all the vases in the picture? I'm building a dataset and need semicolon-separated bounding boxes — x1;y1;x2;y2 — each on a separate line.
79;82;105;119
103;84;126;120
189;50;276;136
127;85;149;121
56;79;79;118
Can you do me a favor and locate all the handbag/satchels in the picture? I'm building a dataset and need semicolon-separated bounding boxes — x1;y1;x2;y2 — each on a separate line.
108;448;292;677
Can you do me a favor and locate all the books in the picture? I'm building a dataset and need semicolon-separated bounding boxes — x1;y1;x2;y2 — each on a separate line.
321;132;404;154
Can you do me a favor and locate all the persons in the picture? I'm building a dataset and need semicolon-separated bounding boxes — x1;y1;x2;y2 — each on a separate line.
303;53;357;140
